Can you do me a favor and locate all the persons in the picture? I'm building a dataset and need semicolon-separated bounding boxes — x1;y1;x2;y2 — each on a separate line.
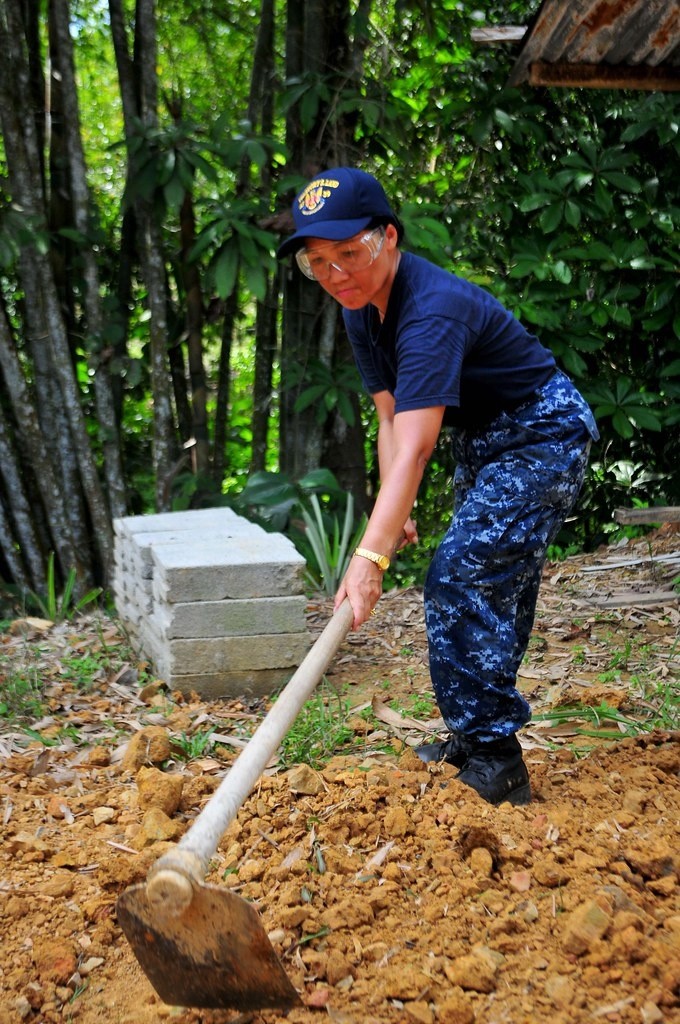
277;169;599;809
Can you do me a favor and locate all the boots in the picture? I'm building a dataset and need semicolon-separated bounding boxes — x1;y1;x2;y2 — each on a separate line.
412;731;521;768
454;748;533;806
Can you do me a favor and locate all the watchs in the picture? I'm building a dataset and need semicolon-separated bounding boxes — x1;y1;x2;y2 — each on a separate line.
353;548;391;573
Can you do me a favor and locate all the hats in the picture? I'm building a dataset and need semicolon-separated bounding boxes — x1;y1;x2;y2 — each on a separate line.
275;166;396;258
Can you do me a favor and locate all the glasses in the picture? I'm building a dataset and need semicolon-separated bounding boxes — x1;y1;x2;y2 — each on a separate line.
295;225;388;280
